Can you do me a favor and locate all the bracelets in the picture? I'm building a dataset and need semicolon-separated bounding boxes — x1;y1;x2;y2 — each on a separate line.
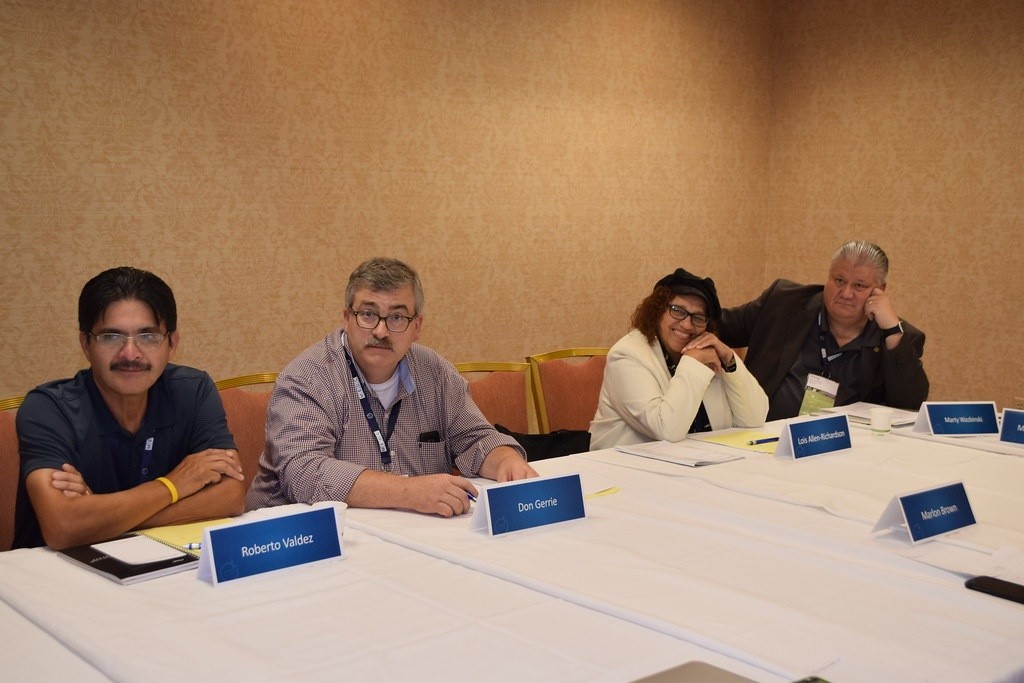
156;477;178;504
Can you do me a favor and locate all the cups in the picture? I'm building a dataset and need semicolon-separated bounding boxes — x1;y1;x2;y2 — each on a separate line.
870;407;891;436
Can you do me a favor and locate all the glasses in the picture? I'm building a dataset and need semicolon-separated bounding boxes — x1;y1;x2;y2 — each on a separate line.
348;305;417;332
667;303;712;327
87;331;169;351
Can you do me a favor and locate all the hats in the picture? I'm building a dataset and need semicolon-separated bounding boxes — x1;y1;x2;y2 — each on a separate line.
654;268;723;318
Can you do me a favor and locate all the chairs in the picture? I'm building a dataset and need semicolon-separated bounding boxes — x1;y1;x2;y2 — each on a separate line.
0;395;26;552
215;372;278;493
524;347;610;435
451;361;541;476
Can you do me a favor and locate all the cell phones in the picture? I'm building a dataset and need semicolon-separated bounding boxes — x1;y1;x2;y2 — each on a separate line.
965;576;1024;604
420;431;440;442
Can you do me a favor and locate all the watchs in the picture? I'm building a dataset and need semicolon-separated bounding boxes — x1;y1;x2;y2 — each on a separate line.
720;360;736;372
881;320;905;339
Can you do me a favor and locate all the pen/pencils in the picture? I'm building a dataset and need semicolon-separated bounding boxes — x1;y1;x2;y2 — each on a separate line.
748;437;781;446
468;492;478;501
182;541;205;550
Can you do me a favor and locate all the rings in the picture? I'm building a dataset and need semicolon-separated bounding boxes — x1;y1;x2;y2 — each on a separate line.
869;301;872;304
85;491;90;495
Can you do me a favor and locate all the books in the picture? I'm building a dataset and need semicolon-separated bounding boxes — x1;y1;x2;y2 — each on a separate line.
821;402;919;427
59;536;199;586
126;516;239;559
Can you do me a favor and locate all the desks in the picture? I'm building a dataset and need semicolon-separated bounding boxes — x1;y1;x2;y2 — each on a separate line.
574;414;1024;585
0;502;786;683
342;455;1024;683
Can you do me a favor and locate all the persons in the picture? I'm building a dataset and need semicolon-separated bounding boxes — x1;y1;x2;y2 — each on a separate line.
12;267;246;551
718;241;930;424
243;258;540;519
587;269;770;453
